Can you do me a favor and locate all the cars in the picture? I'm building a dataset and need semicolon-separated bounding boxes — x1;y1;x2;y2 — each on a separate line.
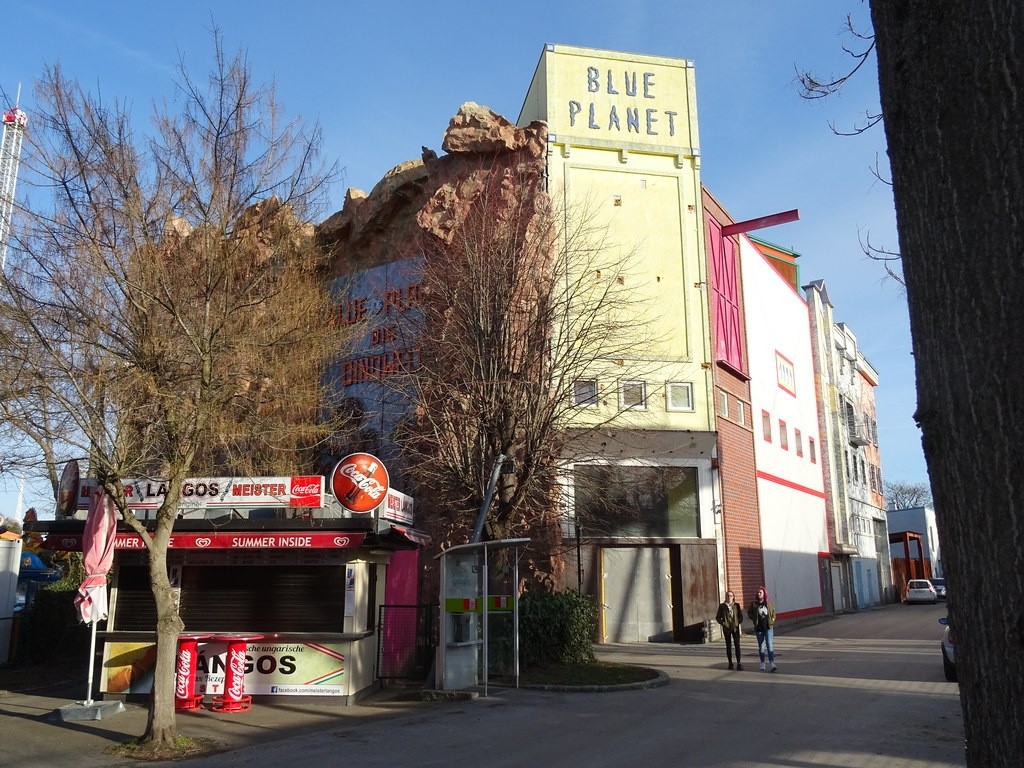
938;610;957;682
906;580;938;604
930;577;946;598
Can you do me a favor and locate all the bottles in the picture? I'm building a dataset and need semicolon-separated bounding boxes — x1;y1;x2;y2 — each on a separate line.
344;461;378;505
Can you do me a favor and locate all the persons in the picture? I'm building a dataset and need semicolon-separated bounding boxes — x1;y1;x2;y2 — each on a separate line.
716;591;744;671
747;587;778;672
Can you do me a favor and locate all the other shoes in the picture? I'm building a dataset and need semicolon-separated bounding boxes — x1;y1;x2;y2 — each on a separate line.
760;662;765;670
728;663;734;670
737;663;744;671
769;663;778;672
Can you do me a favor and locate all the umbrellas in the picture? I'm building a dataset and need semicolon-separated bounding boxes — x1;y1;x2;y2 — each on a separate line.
74;480;118;701
